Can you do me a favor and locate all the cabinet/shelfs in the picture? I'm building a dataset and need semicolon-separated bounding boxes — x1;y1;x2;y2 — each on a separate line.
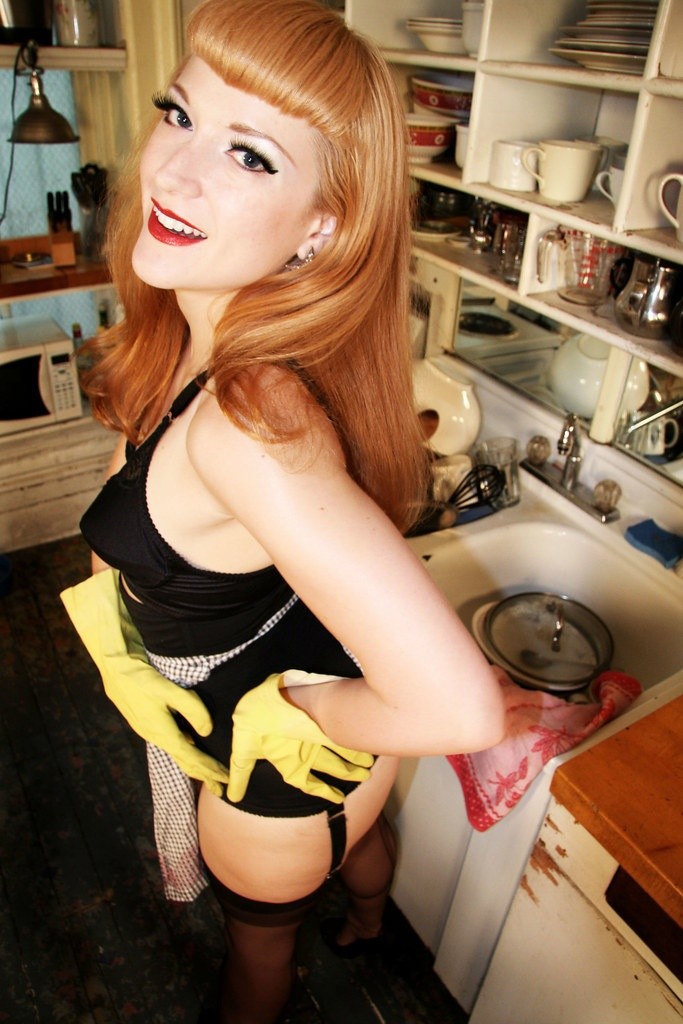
0;0;683;515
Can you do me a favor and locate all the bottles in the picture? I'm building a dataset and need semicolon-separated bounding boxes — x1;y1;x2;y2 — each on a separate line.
73;324;90;401
98;306;111;328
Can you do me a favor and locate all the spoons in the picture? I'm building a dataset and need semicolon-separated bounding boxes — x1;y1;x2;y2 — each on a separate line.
521;650;625;677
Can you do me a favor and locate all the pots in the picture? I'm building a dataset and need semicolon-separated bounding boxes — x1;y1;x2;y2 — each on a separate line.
472;601;597;690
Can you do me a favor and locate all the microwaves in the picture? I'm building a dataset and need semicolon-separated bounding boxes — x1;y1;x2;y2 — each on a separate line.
0;314;82;435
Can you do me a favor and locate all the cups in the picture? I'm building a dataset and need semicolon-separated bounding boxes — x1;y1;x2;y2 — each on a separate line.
462;3;484;58
455;124;468;168
634;415;679;456
476;436;520;510
658;174;683;243
53;1;104;46
431;454;478;513
501;229;527;282
489;136;627;210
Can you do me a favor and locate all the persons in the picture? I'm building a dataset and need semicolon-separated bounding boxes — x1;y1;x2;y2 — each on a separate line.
59;1;507;1024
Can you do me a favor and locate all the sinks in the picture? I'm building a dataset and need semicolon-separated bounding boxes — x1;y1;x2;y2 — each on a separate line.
413;515;683;742
403;470;530;543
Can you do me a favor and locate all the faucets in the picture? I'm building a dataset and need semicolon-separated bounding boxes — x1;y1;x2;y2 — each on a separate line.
556;411;586;495
620;399;683;447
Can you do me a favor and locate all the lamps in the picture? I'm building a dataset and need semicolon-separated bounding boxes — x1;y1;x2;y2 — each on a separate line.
4;1;82;144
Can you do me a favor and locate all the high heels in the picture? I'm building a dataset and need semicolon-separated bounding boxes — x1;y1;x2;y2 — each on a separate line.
319;917;395;968
198;1007;221;1024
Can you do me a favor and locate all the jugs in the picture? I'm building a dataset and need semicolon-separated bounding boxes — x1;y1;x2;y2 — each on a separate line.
610;250;675;339
537;225;625;301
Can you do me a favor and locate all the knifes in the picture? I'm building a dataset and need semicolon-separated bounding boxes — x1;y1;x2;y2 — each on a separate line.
48;191;72;233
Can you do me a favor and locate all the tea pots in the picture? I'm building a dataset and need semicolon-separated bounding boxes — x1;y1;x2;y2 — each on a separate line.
409;178;477;229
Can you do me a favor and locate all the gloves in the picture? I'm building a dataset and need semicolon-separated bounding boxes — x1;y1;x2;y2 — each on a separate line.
225;669;376;805
59;567;228;799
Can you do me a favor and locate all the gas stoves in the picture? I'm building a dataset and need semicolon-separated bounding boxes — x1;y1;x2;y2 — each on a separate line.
453;305;561;359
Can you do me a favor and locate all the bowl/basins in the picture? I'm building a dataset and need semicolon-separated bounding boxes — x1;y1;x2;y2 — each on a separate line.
403;78;473;163
549;334;650;419
412;358;480;455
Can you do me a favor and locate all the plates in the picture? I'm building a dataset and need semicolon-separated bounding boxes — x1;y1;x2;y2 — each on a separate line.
410;223;471;242
548;5;657;75
407;18;466;54
